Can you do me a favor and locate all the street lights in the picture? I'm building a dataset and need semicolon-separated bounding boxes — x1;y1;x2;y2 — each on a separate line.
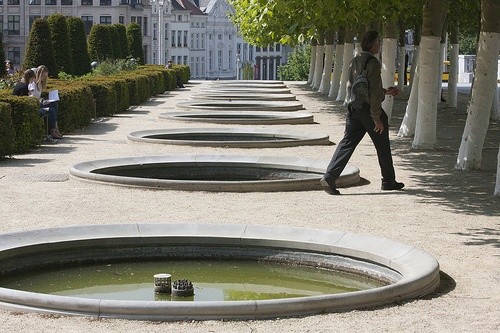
235;55;242;79
150;0;172;65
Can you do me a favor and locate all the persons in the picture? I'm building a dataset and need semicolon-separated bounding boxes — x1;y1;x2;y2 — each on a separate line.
321;30;405;194
12;69;33;96
91;62;97;70
164;59;185;88
28;65;64;139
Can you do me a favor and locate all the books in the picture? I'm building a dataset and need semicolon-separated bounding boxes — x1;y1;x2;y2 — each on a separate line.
46;89;60;102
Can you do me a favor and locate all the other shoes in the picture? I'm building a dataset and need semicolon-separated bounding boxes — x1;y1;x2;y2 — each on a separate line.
381;178;405;190
51;131;63;139
320;176;340;195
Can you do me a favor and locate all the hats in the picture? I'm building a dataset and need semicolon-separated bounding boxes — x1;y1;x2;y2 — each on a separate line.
362;31;379;41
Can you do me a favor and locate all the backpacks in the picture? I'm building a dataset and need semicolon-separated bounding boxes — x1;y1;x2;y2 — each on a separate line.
351;57;370;110
